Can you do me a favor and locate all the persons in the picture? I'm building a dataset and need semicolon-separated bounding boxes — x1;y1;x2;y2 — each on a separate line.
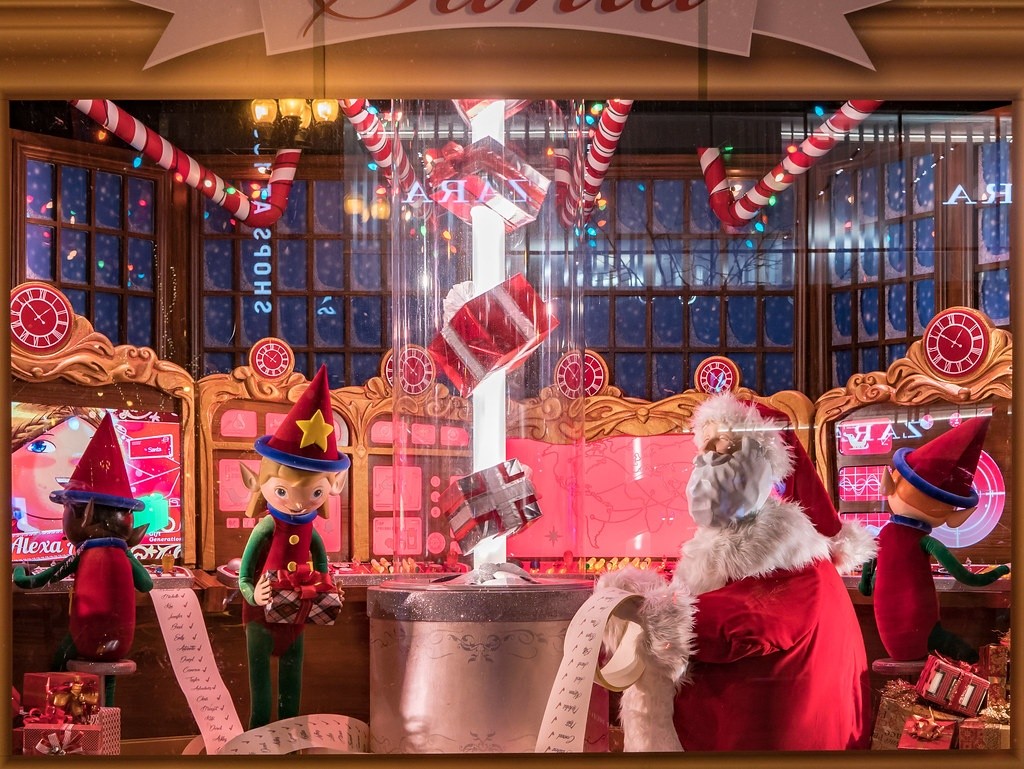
238;364;352;730
858;406;1010;663
13;410;154;710
594;392;876;753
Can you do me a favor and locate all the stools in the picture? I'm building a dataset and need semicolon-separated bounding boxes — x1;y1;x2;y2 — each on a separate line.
66;657;137;706
875;658;926;684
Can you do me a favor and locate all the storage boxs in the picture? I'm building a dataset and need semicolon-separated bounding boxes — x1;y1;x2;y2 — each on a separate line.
13;728;24;754
80;707;121;754
22;722;105;756
979;644;1008;708
437;456;543;555
23;672;102;715
870;699;964;751
897;715;960;753
959;724;1010;749
424;272;560;397
262;569;341;627
453;98;534;128
918;655;992;714
429;135;552;233
964;717;987;723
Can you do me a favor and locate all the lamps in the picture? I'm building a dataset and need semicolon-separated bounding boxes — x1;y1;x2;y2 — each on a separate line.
343;191;390;224
253;98;341;149
